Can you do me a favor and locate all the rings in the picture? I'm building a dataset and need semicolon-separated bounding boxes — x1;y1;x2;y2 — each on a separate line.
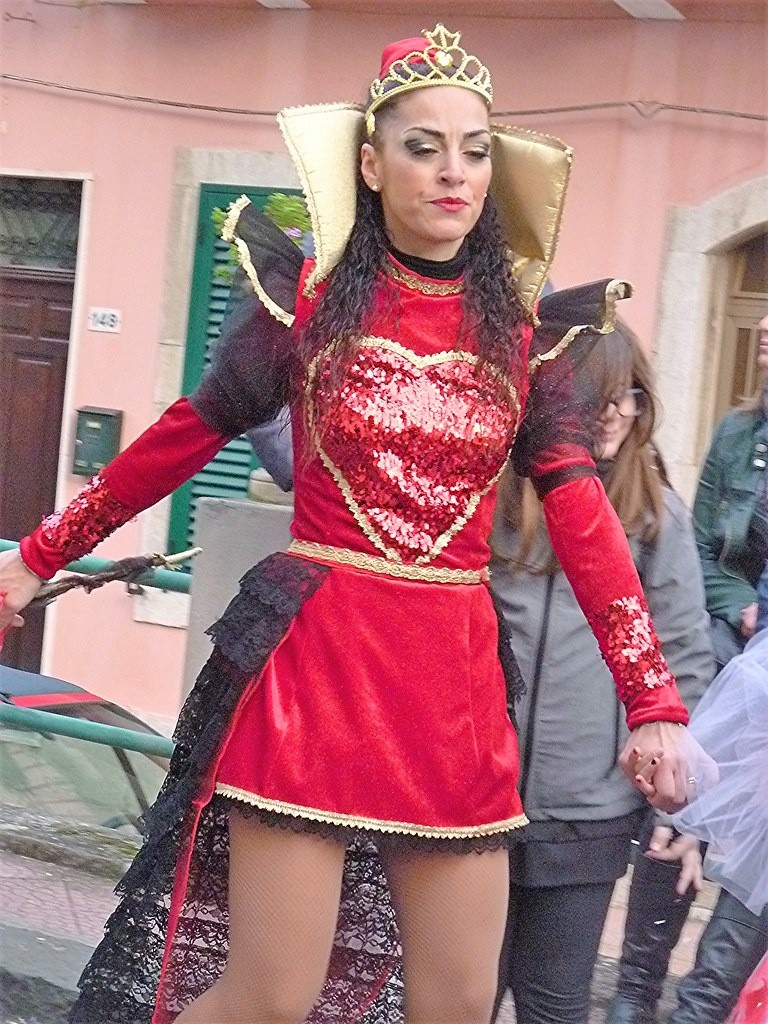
688;778;696;782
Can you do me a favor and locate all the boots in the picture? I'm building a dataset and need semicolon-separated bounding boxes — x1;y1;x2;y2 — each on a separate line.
606;852;698;1024
667;883;768;1024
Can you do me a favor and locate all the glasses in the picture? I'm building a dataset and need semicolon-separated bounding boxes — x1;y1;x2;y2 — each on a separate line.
591;389;648;426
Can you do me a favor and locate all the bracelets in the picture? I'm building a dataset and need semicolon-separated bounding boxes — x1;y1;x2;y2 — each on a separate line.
642;722;683;726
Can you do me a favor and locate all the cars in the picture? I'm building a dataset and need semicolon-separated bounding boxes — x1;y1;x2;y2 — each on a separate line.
0;666;173;1024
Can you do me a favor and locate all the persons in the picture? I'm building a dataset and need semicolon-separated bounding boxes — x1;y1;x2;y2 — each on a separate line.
0;34;718;1023
497;314;768;1024
